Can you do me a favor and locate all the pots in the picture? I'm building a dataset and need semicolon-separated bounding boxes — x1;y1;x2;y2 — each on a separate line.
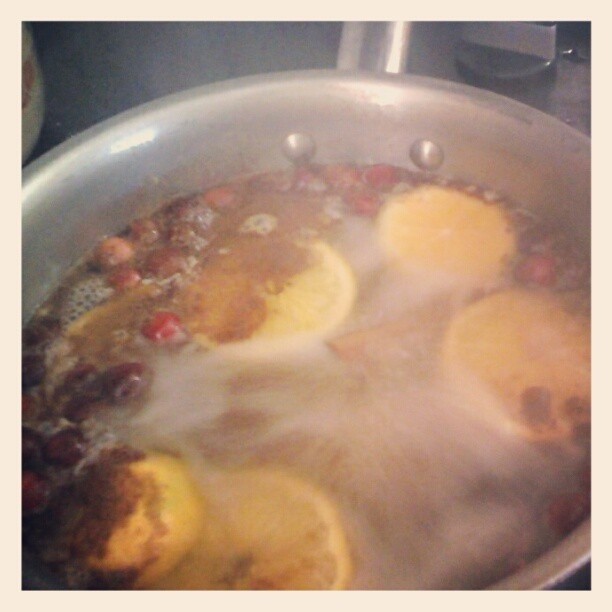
22;21;590;590
